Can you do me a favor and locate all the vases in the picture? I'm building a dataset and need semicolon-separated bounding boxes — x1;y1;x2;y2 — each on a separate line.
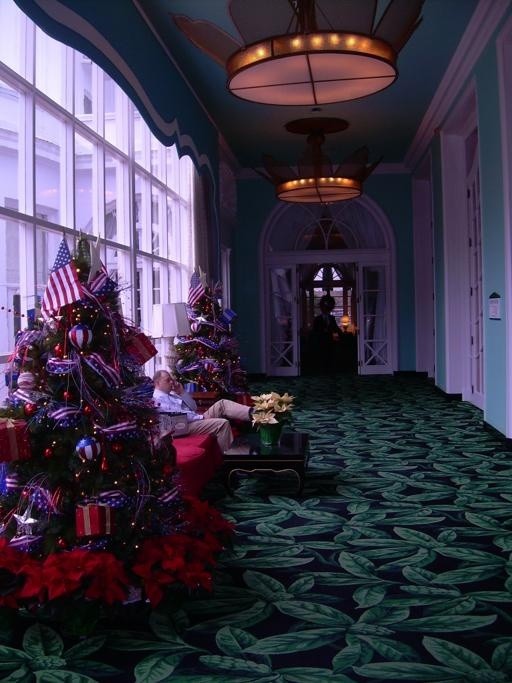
259;424;285;447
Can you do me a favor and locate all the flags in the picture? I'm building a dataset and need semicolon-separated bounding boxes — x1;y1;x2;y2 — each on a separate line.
89;261;118;311
187;270;205;307
40;236;85;327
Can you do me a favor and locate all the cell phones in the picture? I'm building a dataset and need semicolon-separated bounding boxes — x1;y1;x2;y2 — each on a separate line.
171;385;175;390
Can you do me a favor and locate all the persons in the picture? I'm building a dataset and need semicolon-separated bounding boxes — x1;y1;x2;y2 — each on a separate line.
150;369;263;478
313;304;342;374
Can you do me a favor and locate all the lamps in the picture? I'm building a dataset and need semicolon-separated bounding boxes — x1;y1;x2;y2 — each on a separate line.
225;0;401;206
144;302;192;373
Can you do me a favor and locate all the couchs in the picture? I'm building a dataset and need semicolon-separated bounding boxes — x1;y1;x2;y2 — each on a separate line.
145;391;238;497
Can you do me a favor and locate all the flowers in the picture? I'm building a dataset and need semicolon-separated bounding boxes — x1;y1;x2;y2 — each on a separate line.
251;392;300;428
